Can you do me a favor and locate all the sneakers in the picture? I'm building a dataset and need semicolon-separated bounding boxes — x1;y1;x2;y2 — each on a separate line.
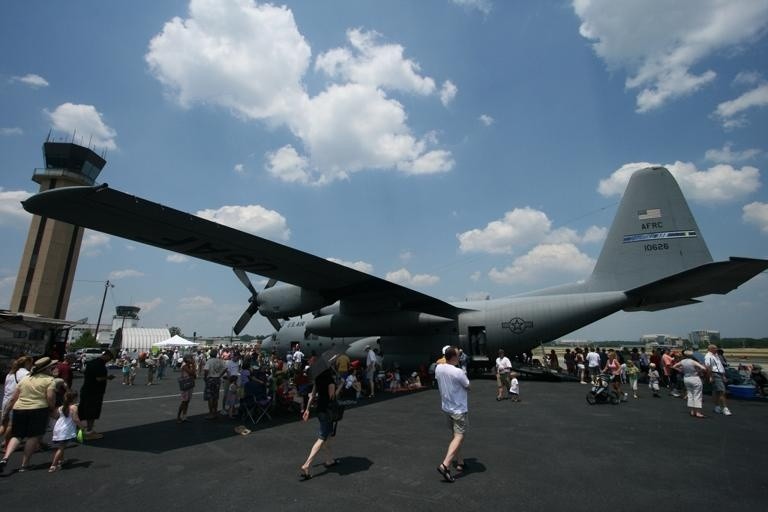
715;405;722;414
85;431;102;441
721;407;732;415
57;460;65;470
0;459;8;475
19;464;37;471
49;465;57;473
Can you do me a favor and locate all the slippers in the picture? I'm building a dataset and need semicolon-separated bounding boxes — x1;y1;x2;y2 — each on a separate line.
300;467;313;479
325;458;340;468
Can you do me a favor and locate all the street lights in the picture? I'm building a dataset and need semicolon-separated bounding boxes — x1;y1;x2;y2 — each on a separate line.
94;281;114;342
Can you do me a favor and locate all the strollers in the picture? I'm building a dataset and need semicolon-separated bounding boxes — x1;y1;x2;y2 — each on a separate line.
584;370;622;404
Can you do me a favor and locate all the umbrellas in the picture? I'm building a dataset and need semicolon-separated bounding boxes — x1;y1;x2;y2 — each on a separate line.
157;334;200;346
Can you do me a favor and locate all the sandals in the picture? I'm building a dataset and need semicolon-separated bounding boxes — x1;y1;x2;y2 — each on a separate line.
437;462;456;482
176;410;239;424
457;462;469;474
690;410;708;418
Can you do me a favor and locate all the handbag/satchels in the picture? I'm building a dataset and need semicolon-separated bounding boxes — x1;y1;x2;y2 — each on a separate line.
178;366;195;392
327;392;344;438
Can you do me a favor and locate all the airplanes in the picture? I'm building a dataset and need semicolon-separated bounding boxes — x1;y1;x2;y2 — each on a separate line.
20;166;768;382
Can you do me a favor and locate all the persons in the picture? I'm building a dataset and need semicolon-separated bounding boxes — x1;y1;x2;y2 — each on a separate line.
545;344;767;418
435;346;471;483
496;349;512;401
508;371;521;402
0;348;117;473
301;367;345;480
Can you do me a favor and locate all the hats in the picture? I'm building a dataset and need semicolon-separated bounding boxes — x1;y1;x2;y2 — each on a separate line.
362;344;370;351
29;356;59;374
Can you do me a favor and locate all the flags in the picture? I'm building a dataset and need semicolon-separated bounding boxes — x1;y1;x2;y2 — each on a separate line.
637;208;662;220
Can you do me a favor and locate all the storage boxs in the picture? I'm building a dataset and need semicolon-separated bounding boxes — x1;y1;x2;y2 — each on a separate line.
726;383;755;400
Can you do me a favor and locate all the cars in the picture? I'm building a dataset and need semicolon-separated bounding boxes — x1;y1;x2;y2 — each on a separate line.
75;348;107;366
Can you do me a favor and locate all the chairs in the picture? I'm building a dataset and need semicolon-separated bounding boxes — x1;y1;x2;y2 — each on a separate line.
239;381;274;427
